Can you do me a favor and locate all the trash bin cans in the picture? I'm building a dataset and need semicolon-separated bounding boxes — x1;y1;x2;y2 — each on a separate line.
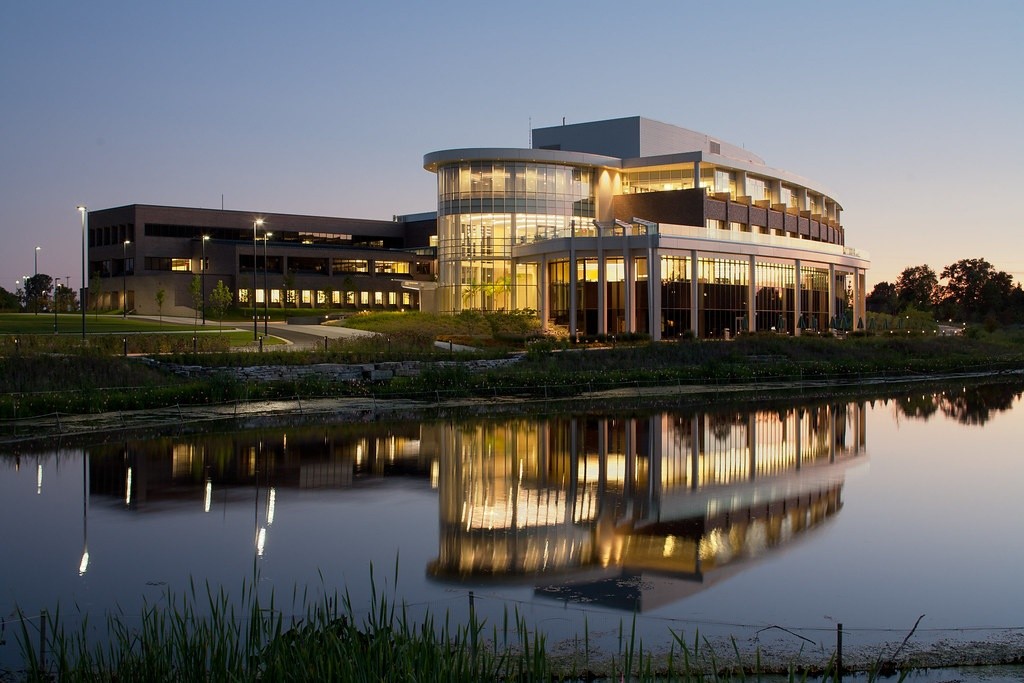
724;328;730;340
833;329;838;337
795;327;801;336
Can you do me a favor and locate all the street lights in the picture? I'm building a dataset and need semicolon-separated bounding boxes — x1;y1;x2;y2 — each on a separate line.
253;219;262;340
35;246;41;316
55;277;60;335
202;236;209;324
264;232;273;336
123;240;130;320
77;206;87;339
15;275;30;311
